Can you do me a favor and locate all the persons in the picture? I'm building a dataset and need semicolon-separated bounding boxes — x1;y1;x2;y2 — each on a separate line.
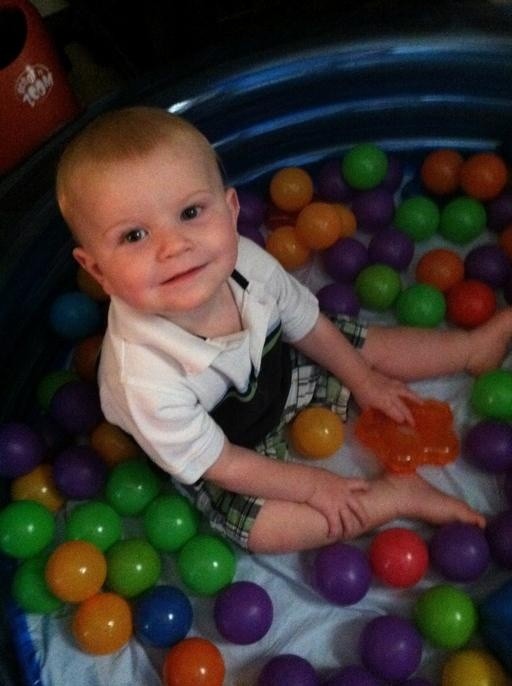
54;104;512;553
